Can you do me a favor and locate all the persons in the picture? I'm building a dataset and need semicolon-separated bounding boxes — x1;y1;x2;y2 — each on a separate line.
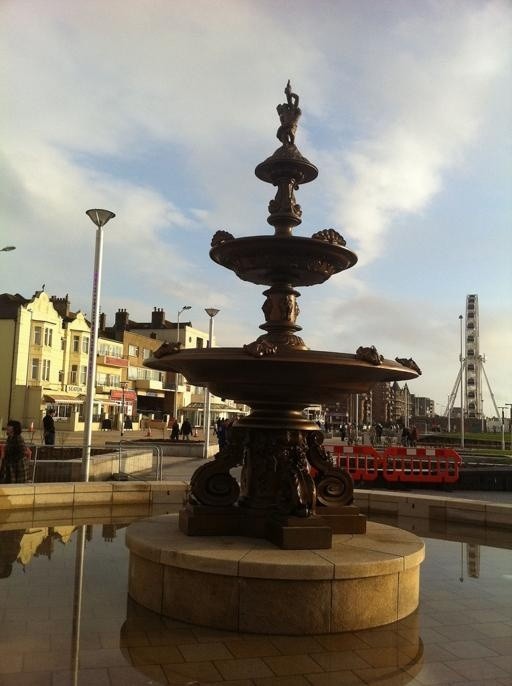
43;409;57;445
181;418;193;440
1;420;30;483
170;420;180;439
212;415;244;435
1;528;26;580
314;419;457;448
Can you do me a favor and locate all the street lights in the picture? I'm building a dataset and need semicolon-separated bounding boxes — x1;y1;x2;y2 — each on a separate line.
120;381;129;435
82;208;115;483
173;306;192;419
203;308;220;459
